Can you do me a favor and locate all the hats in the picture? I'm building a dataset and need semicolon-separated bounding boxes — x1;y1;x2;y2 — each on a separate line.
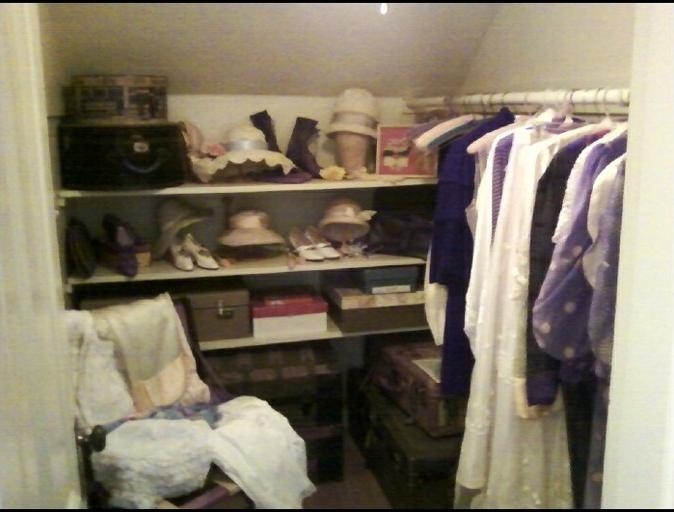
216;210;285;247
325;86;381;140
205;123;297;179
148;197;215;261
319;197;377;242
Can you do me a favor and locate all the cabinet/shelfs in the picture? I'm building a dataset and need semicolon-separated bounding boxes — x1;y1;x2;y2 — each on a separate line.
55;177;436;349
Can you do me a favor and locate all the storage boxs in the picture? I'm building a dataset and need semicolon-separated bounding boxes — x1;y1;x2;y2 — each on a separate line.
249;282;331;340
185;277;251;342
354;265;419;293
348;340;471;512
320;274;429;330
72;73;169;122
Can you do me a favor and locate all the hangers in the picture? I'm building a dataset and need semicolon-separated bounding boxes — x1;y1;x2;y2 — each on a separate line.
404;89;630;154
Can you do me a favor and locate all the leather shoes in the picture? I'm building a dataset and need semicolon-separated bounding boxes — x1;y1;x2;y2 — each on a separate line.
287;223;340;261
169;234;219;271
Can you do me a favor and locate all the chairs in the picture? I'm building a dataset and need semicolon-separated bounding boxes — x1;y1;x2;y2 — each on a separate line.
61;289;321;511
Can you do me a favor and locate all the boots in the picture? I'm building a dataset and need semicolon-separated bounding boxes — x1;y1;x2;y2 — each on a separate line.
249;109;282;153
285;116;325;179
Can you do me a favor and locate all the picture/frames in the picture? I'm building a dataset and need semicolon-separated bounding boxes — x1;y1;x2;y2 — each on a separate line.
375;122;438;179
